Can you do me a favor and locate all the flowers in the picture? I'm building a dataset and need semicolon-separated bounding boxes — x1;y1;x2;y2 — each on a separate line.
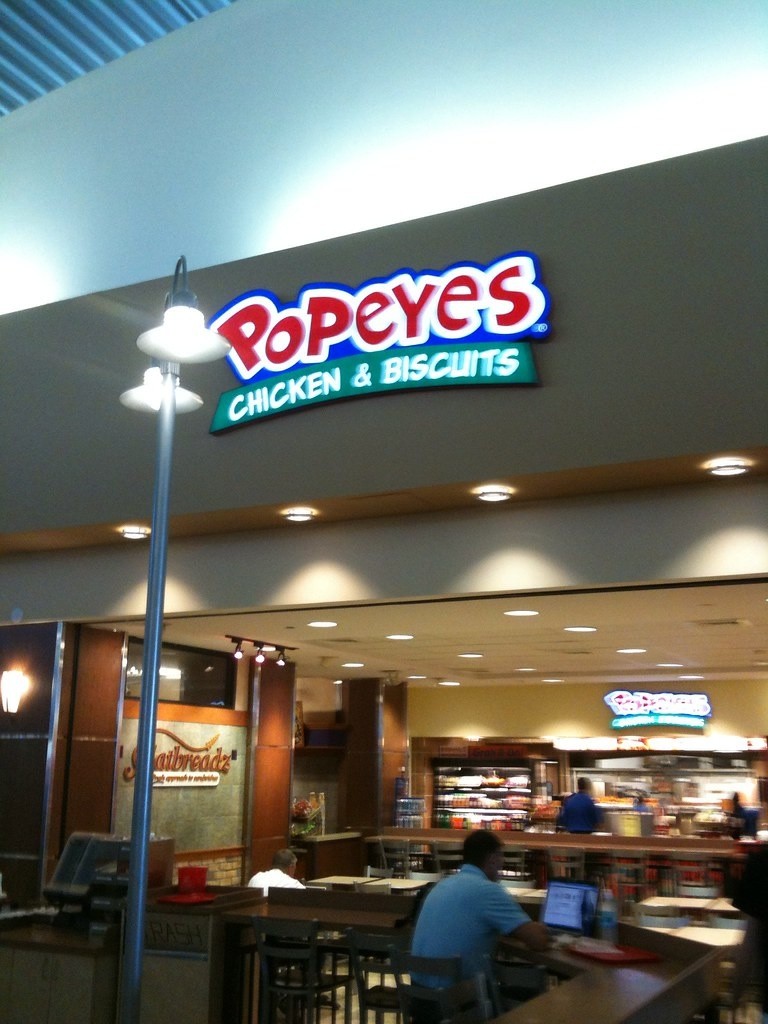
292;799;312;817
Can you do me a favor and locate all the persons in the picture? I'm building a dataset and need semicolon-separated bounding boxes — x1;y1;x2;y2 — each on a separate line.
732;791;747;840
555;776;606;880
246;849;341;1011
735;846;768;1024
631;795;654;812
408;829;560;1013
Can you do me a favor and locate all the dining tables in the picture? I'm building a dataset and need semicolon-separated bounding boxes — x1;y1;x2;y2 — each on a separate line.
364;865;395;879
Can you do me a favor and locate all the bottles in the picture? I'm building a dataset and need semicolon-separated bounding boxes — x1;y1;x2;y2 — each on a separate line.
437;793;467;807
435;812;472;829
599;889;619;944
310;792;318;808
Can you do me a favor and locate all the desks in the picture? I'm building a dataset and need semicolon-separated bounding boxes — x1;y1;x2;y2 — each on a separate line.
308;874;380;891
362;878;428;896
633;895;740;917
506;885;548;904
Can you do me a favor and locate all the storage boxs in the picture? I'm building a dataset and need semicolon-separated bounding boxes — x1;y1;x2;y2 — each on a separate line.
608;812;655;835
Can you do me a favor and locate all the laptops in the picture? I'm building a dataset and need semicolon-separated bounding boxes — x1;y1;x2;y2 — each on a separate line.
538;877;601;939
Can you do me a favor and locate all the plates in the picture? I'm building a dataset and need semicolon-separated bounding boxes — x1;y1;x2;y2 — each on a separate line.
158;893;218;905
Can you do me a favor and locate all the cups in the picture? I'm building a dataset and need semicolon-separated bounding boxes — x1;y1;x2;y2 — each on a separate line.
179;867;208;896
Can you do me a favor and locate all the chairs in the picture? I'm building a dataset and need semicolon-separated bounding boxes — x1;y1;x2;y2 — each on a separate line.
343;950;401;1024
497;844;526;881
479;954;557;1016
432;839;464;873
388;944;461;1024
543;844;586;881
404;868;443;896
353;881;391;895
378;837;412;879
251;913;353;1024
635;909;690;927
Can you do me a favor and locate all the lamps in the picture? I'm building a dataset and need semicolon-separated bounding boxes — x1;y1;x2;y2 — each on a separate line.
231;639;243;660
274;646;286;666
253;641;264;663
